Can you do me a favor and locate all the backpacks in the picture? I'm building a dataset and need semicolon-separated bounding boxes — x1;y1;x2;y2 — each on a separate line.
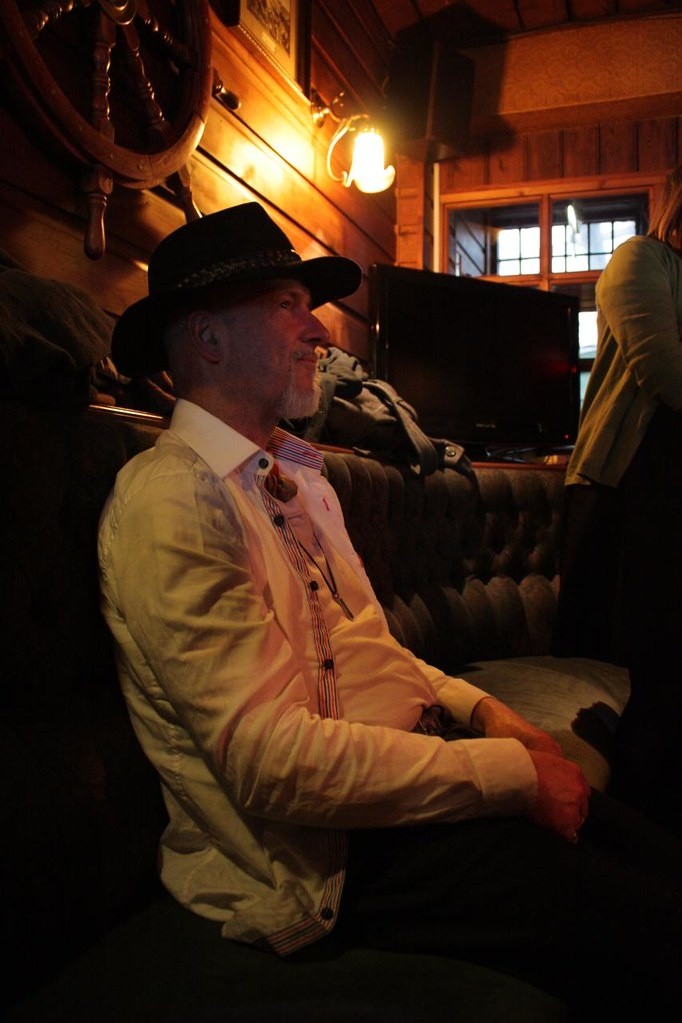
308;343;437;475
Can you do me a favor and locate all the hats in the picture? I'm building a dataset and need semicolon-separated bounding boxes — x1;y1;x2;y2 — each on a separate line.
112;201;362;381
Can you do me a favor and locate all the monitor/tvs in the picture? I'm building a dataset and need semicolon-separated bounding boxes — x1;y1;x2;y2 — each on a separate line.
366;263;581;460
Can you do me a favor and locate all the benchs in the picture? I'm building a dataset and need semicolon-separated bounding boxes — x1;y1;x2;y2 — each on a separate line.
0;399;682;1023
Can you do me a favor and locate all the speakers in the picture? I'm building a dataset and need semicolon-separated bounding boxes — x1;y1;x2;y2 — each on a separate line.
391;42;475;162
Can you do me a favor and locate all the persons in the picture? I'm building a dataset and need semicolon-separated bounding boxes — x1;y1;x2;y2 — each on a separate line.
96;202;682;1023
563;166;682;830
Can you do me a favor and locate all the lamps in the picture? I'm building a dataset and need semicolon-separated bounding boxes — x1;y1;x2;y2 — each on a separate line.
312;104;396;193
